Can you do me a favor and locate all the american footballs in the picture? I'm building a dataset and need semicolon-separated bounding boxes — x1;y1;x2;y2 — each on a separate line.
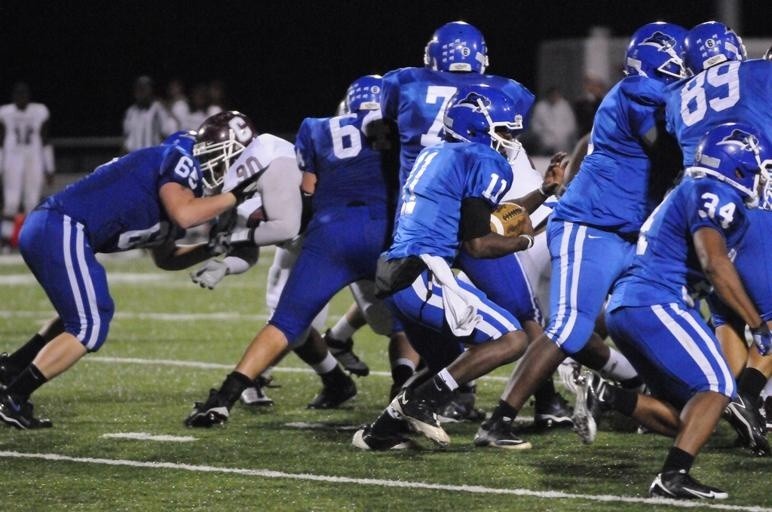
232;202;267;228
490;202;525;237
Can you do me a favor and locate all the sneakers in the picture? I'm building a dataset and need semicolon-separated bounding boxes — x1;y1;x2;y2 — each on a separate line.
559;353;656;447
649;469;729;500
320;328;369;376
725;392;770;456
308;376;356;409
475;414;533;450
530;393;575;429
238;387;273;408
352;377;482;450
0;353;53;429
184;402;229;427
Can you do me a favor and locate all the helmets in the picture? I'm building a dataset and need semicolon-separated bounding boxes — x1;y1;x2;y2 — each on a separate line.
336;75;386;114
693;122;772;211
425;21;487;74
624;21;745;84
443;85;523;166
193;112;257;190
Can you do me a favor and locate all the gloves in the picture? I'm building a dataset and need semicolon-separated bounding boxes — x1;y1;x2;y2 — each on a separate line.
190;260;228;289
209;207;253;255
750;321;772;355
230;167;271;203
